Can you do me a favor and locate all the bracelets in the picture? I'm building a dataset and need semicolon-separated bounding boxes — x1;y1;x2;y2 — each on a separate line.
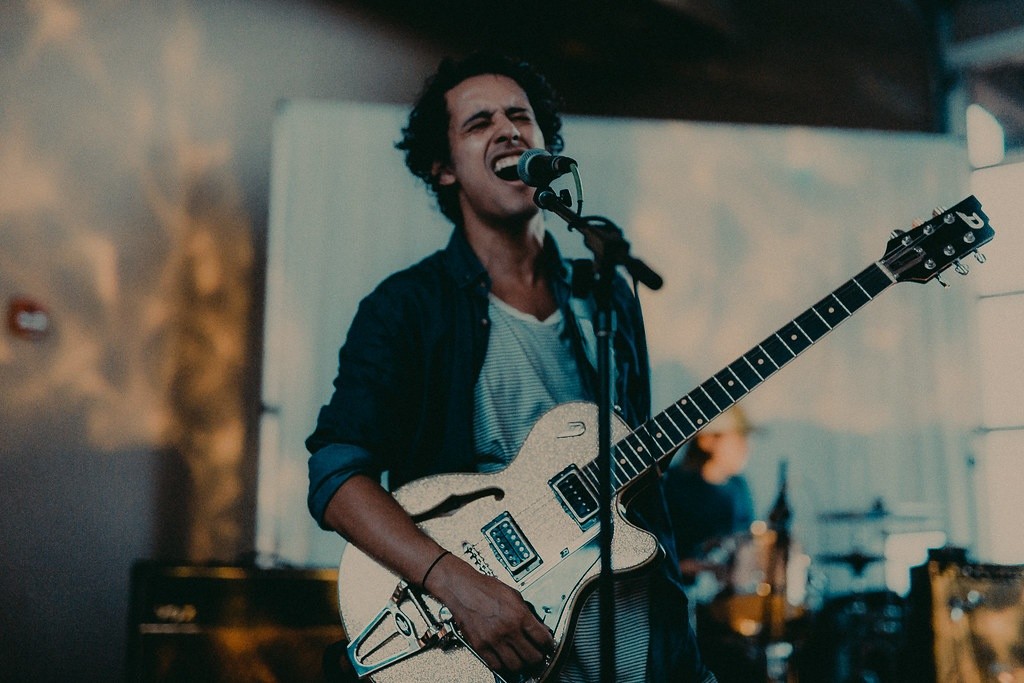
422;551;452;591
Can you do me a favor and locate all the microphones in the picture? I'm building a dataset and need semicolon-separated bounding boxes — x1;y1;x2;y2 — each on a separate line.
517;148;578;187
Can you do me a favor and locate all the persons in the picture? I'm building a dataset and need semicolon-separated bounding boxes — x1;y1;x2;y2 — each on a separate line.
305;55;719;683
660;407;760;631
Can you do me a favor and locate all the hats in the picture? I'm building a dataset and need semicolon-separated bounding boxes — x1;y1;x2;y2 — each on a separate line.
695;404;759;440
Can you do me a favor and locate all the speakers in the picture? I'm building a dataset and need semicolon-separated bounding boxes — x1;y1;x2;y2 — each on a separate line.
124;559;374;683
909;561;1024;683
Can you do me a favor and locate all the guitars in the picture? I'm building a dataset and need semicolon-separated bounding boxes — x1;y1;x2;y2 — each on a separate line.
336;190;997;683
